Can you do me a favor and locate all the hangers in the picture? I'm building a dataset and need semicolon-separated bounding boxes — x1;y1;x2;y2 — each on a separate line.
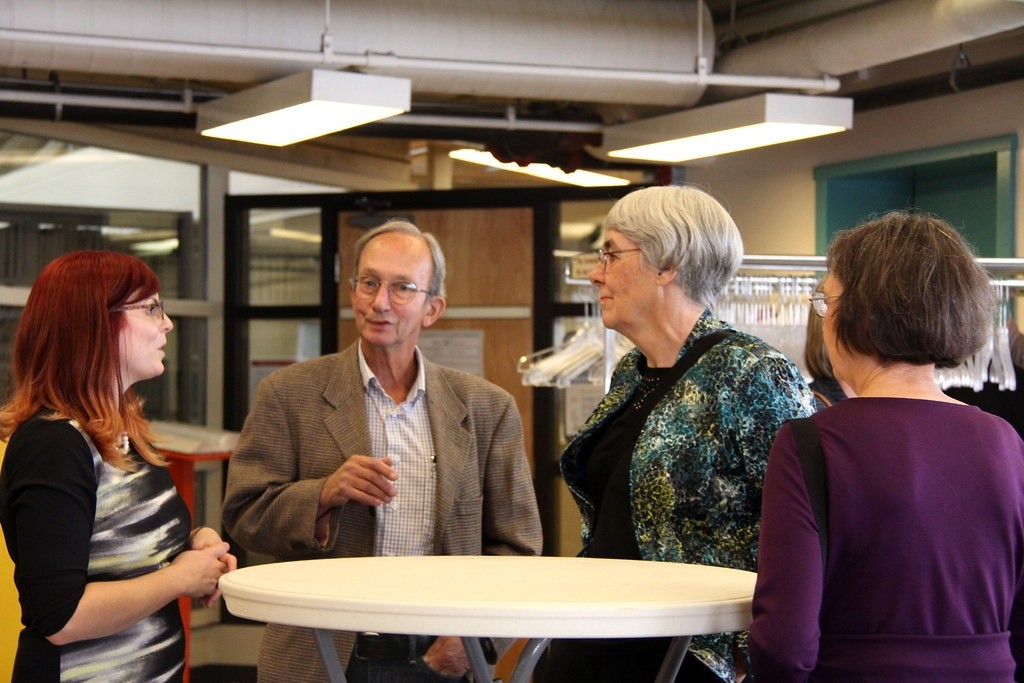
512;269;1019;395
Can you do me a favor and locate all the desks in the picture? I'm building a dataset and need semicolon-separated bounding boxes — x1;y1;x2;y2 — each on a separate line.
220;557;756;683
142;423;243;683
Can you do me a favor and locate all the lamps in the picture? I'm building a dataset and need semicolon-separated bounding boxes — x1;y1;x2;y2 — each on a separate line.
198;67;411;147
604;92;852;161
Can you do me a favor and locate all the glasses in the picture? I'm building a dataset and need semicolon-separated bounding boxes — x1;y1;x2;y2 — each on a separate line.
354;272;432;300
809;291;842;318
598;248;642;264
108;298;164;320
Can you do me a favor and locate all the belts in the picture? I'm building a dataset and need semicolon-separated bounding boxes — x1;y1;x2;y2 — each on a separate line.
353;633;437;662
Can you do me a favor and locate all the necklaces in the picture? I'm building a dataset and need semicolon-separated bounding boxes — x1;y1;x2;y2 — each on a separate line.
111;431;130;455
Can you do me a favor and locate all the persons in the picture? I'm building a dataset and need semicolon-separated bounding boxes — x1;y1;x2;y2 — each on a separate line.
220;222;543;683
532;186;817;683
749;213;1024;683
0;251;237;683
